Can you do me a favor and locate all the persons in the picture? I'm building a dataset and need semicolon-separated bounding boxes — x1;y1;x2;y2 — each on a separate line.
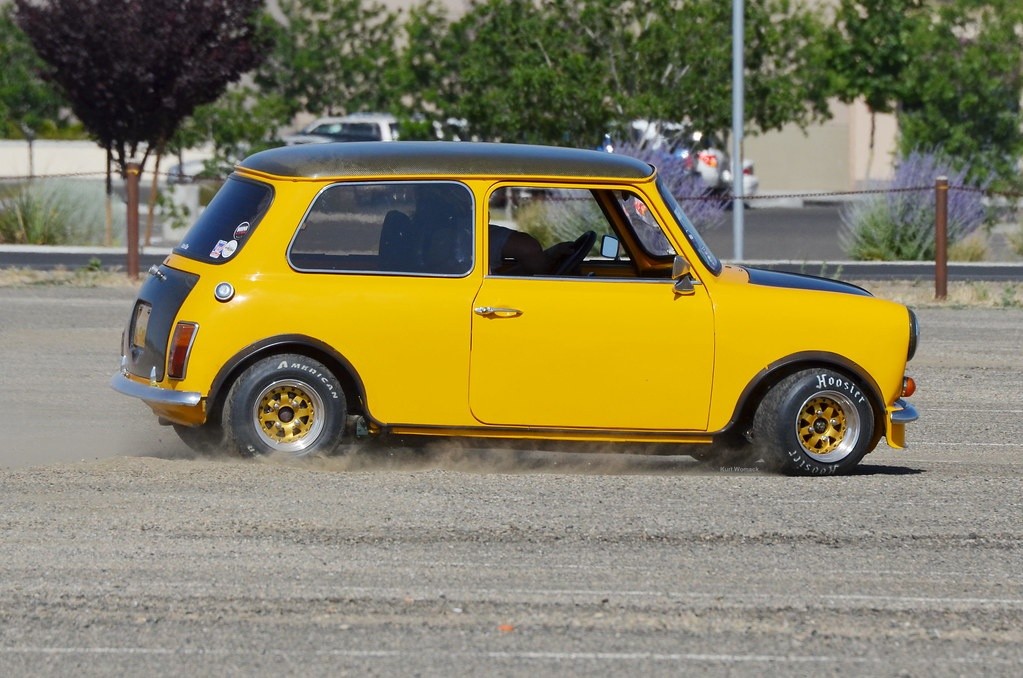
432;199;542;274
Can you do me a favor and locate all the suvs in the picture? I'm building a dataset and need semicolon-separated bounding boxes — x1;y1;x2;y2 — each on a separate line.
286;116;461;143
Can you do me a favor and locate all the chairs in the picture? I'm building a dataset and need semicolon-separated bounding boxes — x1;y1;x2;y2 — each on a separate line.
380;212;435;273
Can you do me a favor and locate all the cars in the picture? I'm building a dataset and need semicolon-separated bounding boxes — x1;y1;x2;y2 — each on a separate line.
107;139;922;477
167;154;244;183
592;126;760;208
